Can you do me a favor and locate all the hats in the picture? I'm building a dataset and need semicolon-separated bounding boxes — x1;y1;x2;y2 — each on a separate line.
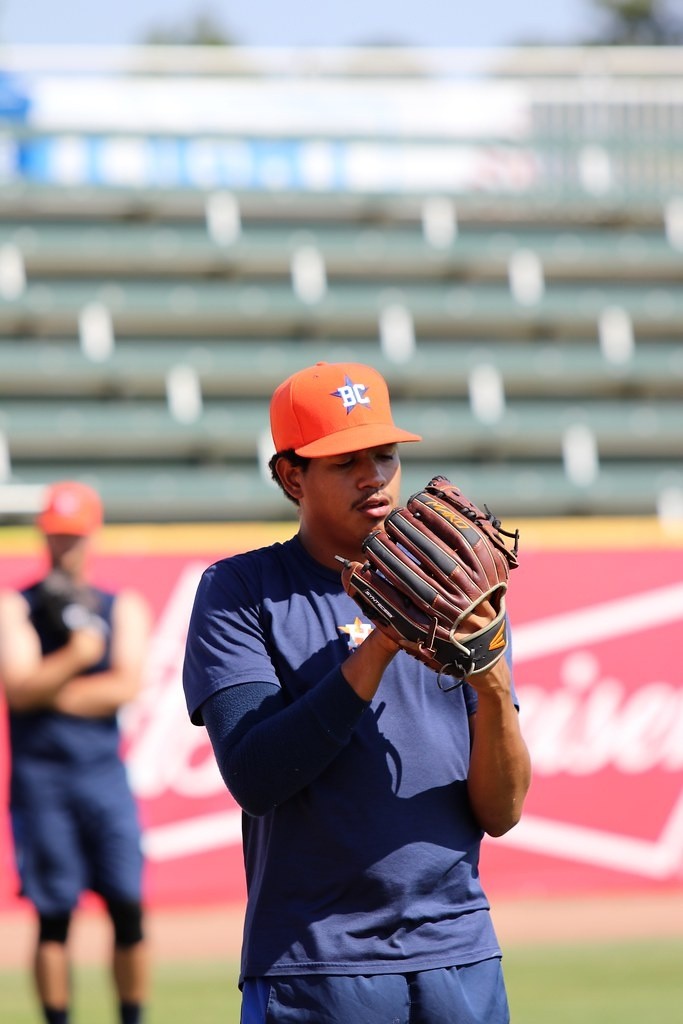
35;479;103;540
267;358;423;461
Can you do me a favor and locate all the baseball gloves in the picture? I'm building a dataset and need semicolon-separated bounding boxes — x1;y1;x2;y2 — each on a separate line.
340;475;511;676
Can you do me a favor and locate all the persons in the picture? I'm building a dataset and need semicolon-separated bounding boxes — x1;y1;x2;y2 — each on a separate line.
0;482;151;1024
181;362;532;1024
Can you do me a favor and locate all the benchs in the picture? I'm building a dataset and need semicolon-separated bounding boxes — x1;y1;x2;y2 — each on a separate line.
0;173;681;526
